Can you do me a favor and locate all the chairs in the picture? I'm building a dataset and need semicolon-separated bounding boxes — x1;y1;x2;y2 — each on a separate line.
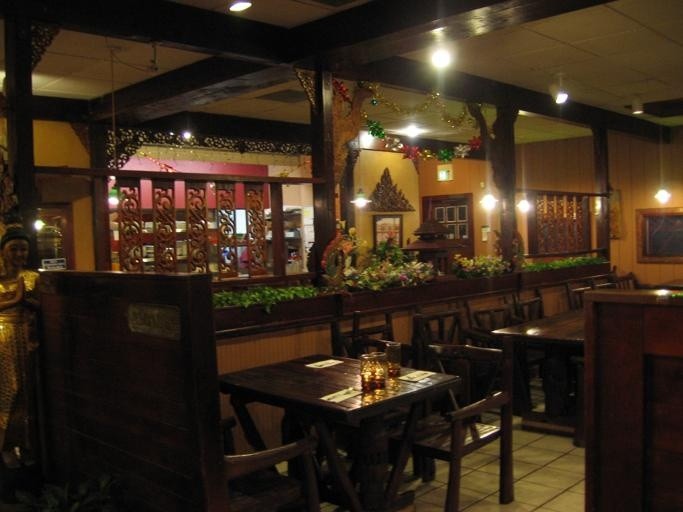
212;304;515;508
410;264;642;439
1;450;20;469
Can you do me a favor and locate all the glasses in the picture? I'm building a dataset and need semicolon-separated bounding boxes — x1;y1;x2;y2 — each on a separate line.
348;125;373;212
547;68;570;110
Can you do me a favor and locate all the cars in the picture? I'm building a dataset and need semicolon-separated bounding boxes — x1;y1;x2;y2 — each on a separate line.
387;342;402;377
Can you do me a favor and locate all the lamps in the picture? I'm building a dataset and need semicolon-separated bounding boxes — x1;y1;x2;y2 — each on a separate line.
348;125;373;212
547;68;570;110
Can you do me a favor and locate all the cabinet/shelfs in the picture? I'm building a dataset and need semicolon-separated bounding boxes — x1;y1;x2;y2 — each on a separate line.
360;351;386;392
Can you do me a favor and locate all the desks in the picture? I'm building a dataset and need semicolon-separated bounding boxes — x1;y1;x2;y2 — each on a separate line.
582;287;683;510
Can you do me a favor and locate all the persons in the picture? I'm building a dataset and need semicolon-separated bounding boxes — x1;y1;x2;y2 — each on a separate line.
0;223;41;472
